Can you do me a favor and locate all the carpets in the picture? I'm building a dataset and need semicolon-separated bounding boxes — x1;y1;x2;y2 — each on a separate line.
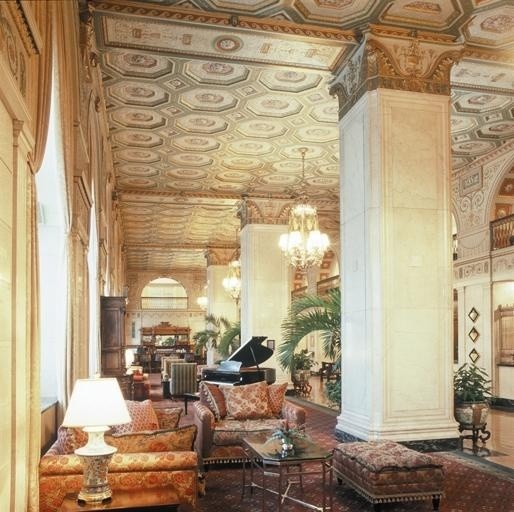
149;397;514;512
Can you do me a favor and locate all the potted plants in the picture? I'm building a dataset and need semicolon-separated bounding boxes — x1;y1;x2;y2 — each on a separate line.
290;349;318;383
454;364;493;429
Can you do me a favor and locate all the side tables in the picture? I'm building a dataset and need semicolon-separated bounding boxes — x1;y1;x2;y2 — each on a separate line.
59;485;180;512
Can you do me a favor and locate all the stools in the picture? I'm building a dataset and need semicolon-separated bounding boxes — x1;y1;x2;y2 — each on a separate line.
334;438;446;511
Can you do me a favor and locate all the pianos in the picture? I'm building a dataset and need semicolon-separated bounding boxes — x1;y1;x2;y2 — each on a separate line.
196;336;276;386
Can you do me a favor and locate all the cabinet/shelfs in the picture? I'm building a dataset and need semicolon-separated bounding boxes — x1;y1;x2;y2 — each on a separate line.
101;297;129;377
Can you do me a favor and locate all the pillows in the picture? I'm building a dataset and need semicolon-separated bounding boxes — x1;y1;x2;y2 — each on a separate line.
112;399;159;433
218;381;276;420
268;382;289;419
200;381;226;421
153;407;183;430
57;424;198;454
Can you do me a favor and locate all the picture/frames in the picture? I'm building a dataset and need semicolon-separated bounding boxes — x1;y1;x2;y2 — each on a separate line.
267;340;275;350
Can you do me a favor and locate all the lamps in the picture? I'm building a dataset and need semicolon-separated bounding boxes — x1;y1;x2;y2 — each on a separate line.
60;378;133;506
278;147;330;271
222;225;241;307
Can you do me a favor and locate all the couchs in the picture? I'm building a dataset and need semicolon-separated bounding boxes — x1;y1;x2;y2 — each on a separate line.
160;357;178;387
169;363;198;415
39;408;198;512
161;359;186;398
193;399;307;472
